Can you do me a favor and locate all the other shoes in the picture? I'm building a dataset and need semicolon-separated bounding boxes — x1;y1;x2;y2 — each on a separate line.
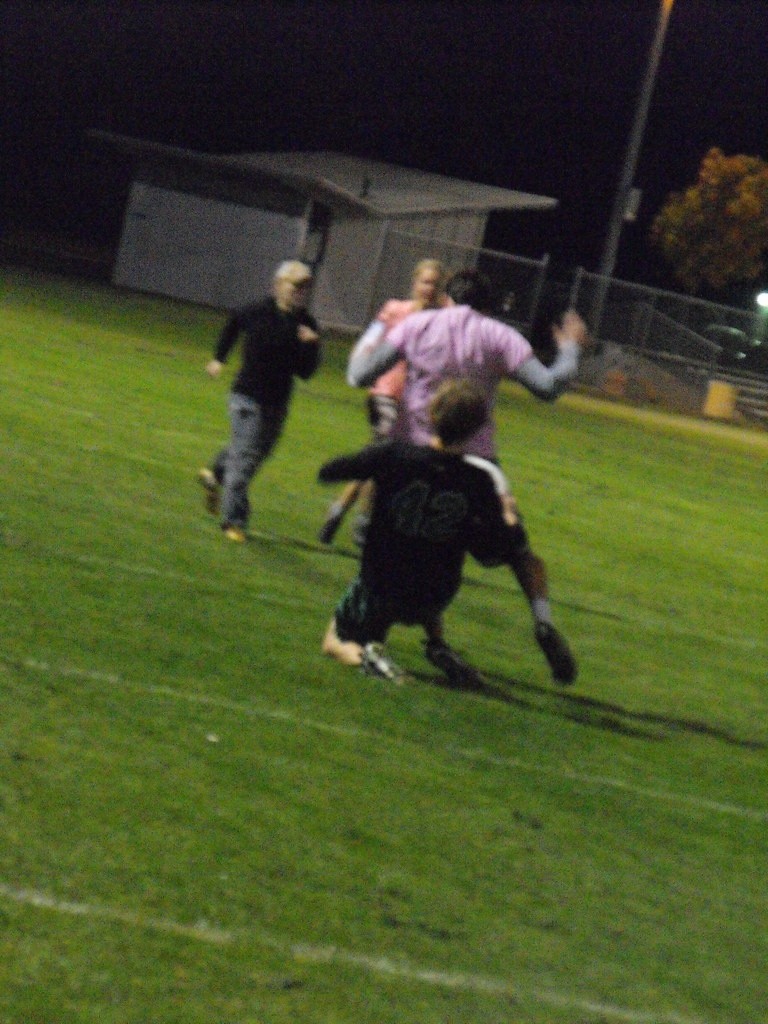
221;525;249;546
420;639;489;693
534;620;576;686
195;468;221;511
353;516;370;546
318;508;340;545
359;642;416;687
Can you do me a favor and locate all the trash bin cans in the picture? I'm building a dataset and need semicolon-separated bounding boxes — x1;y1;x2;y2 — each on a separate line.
703;379;739;420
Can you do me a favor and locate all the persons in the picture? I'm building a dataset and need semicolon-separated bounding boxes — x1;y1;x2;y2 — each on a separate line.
313;260;585;690
193;257;320;548
